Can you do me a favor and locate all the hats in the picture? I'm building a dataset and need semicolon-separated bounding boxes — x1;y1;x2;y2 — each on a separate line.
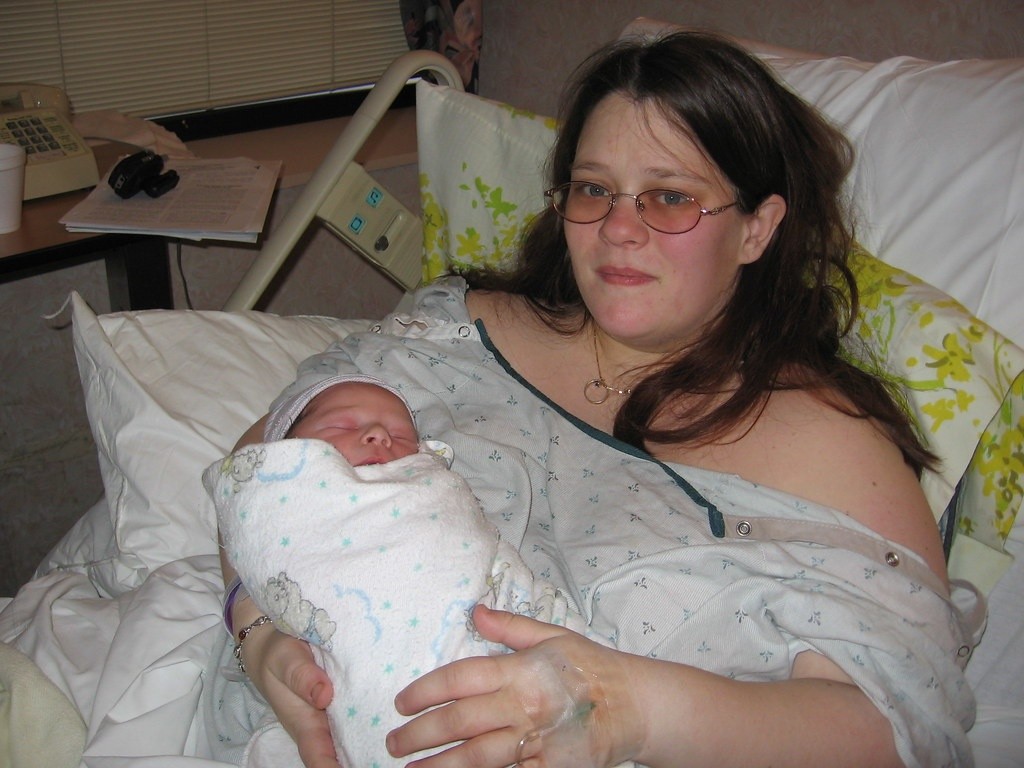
262;373;416;443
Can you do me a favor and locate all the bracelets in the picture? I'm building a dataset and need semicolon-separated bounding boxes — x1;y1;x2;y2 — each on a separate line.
221;574;251;639
231;614;273;675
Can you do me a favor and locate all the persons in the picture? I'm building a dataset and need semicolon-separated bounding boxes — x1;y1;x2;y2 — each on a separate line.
235;349;622;768
209;30;974;768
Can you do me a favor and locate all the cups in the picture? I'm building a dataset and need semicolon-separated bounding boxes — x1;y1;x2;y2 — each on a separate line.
0;144;27;235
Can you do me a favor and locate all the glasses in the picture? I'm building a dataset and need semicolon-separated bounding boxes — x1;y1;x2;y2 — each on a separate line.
545;181;740;235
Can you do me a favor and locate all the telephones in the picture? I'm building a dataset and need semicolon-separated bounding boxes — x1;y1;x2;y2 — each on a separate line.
0;82;101;201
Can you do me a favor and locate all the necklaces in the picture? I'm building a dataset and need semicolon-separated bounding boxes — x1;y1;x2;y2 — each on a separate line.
583;318;635;406
